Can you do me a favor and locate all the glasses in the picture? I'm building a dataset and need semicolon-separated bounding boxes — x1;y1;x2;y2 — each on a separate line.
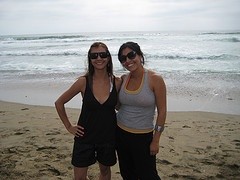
119;50;140;63
89;51;109;58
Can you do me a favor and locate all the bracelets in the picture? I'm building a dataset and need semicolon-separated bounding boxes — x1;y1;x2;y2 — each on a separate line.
154;125;164;132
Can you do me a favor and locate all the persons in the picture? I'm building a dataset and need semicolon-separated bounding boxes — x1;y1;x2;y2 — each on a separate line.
55;42;123;180
115;42;167;180
